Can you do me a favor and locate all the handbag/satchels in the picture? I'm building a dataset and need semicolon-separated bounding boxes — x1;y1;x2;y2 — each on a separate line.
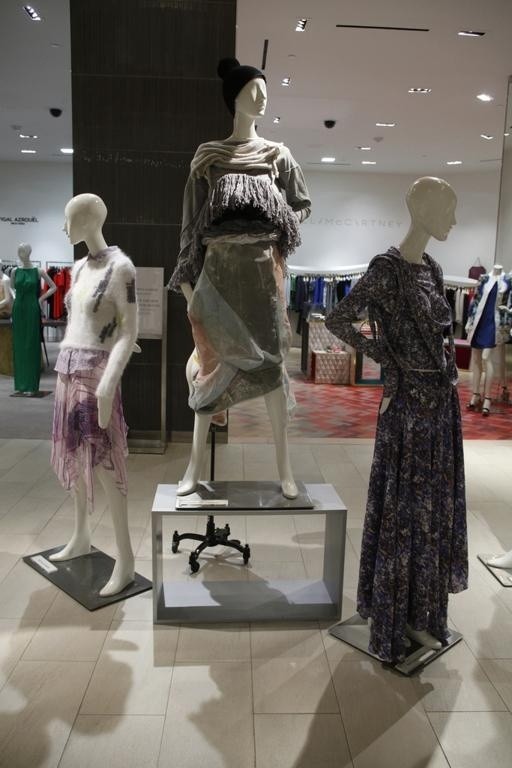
468;257;485;279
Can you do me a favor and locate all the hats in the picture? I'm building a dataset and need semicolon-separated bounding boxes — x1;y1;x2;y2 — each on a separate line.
217;57;267;116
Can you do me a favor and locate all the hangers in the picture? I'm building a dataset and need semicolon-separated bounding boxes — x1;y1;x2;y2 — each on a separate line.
3;263;73;279
288;272;368;285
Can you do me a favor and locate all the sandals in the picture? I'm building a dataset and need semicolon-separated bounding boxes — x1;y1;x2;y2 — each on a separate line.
481;398;491;415
465;392;481;410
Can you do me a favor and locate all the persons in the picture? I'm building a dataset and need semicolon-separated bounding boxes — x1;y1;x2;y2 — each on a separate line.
324;175;467;670
465;265;512;415
166;57;300;500
10;242;56;395
50;194;134;596
0;258;11;313
